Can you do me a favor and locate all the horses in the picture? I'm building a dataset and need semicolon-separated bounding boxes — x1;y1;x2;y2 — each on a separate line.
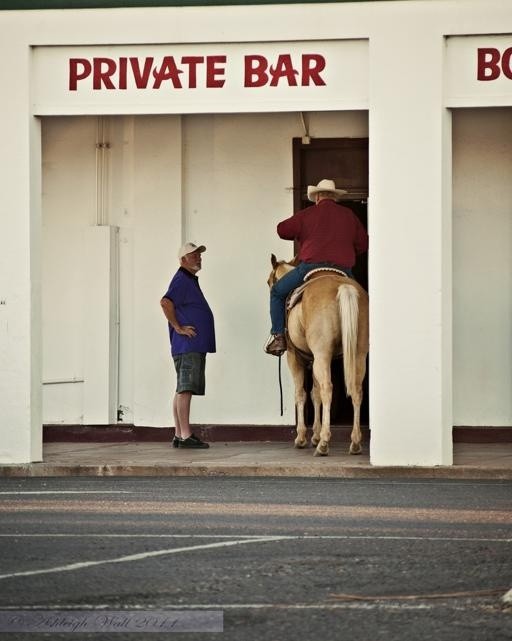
266;252;368;457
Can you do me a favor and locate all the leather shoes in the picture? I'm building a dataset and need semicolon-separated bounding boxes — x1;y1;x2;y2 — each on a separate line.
265;335;287;352
171;435;179;448
177;432;209;449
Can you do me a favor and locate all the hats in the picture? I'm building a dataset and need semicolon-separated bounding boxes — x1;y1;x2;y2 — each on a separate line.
306;179;346;203
178;241;206;262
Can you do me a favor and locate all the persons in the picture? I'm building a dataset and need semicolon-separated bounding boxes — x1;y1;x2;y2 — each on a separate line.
262;178;367;356
160;242;217;450
